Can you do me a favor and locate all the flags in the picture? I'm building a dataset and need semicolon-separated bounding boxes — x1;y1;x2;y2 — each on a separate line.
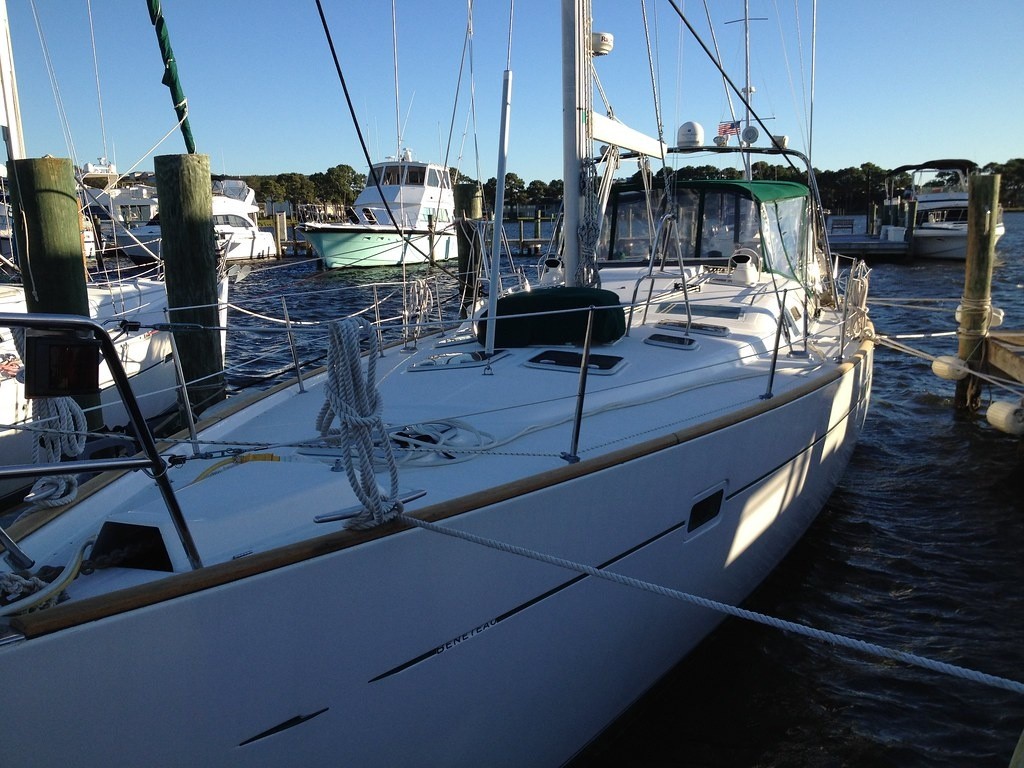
718;121;741;135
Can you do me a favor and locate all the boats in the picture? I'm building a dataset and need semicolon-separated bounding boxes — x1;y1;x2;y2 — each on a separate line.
826;160;1005;262
0;0;877;768
